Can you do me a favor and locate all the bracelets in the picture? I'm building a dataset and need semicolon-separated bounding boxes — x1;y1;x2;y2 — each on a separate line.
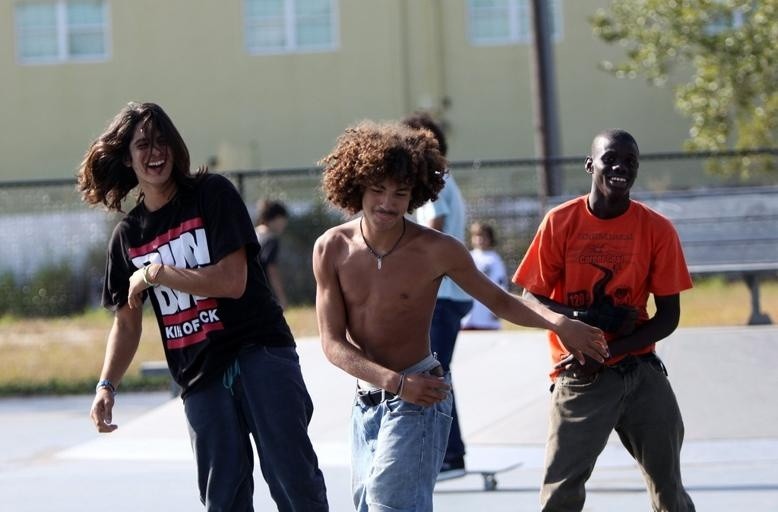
392;376;404;397
572;308;579;319
95;379;115;395
142;263;164;287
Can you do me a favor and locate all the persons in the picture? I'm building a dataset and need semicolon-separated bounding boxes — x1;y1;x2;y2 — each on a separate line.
460;221;507;331
513;129;694;512
75;100;329;512
305;127;609;512
399;111;474;482
254;196;289;311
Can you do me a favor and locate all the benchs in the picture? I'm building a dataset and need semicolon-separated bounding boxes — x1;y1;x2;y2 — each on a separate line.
541;185;778;327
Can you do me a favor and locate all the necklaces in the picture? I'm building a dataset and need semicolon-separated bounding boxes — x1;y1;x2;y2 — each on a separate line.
359;213;407;270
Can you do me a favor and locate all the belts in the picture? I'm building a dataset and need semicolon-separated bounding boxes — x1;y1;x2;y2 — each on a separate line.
356;366;446;409
603;352;654;372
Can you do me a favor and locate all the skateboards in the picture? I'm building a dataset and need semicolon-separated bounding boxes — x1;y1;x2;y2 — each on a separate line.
437;459;524;492
